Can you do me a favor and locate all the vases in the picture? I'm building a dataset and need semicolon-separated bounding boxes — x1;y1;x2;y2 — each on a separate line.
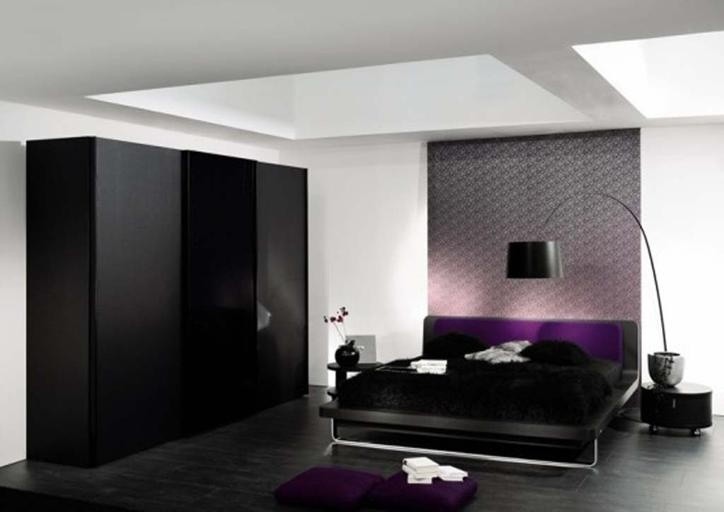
336;340;359;366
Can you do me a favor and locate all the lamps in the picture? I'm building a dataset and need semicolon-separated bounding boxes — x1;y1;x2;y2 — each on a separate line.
506;191;685;387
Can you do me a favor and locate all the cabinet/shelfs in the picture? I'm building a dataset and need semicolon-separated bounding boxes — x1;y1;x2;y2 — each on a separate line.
26;136;182;469
183;150;310;441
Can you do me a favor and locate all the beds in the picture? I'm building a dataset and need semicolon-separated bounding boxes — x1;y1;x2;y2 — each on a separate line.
319;316;641;469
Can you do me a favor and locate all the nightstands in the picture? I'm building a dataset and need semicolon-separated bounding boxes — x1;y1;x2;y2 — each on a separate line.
640;382;713;436
327;362;380;397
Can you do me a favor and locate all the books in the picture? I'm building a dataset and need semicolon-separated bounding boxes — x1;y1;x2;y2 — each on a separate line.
400;456;468;485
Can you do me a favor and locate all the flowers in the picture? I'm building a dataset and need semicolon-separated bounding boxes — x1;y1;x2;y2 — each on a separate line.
323;306;349;344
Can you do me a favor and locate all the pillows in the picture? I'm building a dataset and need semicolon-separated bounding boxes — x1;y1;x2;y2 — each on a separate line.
365;468;480;512
273;465;385;511
423;333;488;356
515;338;591;367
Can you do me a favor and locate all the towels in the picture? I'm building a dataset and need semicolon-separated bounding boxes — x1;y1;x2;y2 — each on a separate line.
401;457;469;484
